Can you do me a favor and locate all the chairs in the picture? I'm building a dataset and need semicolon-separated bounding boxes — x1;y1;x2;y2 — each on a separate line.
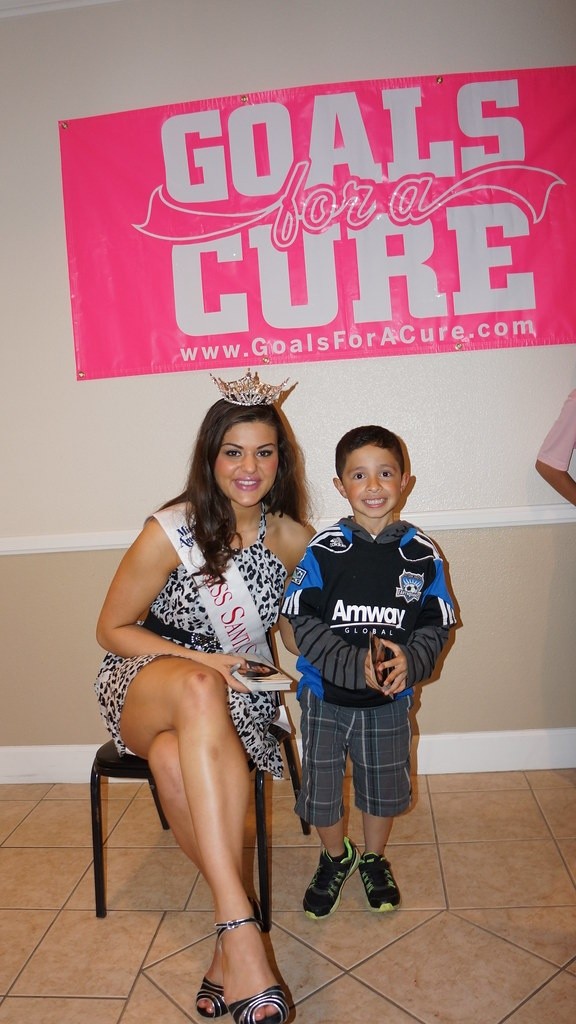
88;628;312;932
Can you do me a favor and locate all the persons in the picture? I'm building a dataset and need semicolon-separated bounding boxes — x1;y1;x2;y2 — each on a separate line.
94;396;321;1023
282;423;458;919
370;634;390;688
240;659;278;678
534;385;576;507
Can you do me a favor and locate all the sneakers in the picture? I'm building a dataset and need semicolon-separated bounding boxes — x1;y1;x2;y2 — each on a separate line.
358;851;403;913
300;837;361;919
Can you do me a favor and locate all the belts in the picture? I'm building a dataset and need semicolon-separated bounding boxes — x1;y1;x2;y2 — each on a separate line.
143;610;269;651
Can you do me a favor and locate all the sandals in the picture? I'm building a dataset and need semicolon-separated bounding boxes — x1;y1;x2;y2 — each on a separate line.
195;898;262;1019
214;916;289;1024
